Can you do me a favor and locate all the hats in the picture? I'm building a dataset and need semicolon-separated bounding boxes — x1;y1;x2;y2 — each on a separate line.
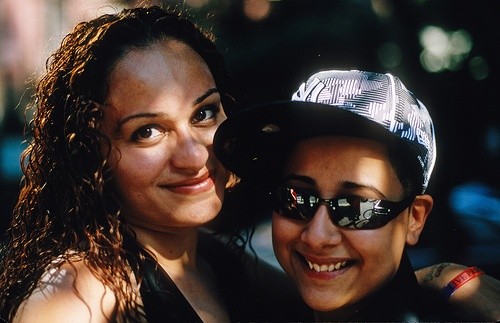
215;70;436;195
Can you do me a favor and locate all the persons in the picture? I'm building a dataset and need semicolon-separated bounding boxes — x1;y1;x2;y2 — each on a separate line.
0;2;500;322
211;69;490;323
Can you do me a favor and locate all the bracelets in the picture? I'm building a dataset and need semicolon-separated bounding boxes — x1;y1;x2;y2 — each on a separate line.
441;264;487;304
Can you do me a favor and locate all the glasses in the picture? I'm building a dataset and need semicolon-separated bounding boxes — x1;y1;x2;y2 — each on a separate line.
267;188;413;230
